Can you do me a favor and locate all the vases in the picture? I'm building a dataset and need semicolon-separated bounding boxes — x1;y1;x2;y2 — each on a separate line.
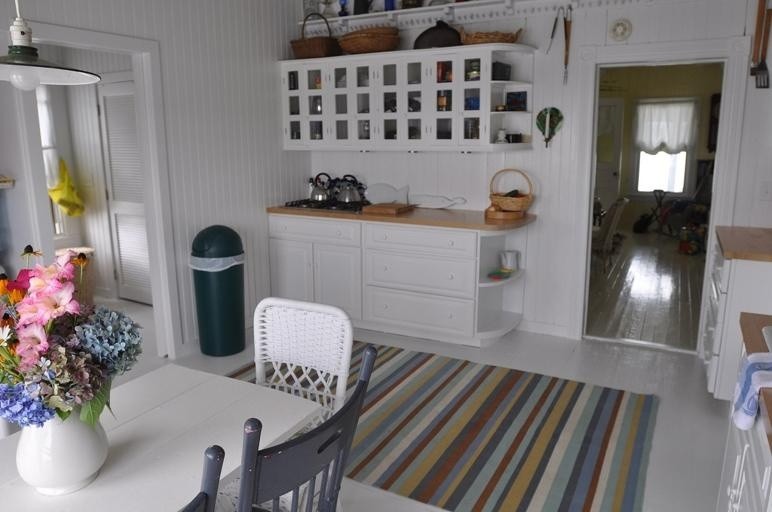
15;405;110;495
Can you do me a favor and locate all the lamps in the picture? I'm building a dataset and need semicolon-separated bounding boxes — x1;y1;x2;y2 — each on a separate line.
0;0;102;91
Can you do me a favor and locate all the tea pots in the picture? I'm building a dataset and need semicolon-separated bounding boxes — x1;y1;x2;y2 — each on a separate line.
311;173;337;202
322;175;363;203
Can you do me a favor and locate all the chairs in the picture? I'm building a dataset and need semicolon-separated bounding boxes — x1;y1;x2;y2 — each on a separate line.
220;297;353;511
591;198;629;274
239;346;377;512
179;446;225;512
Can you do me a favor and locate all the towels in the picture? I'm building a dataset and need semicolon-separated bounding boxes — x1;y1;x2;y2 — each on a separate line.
732;352;772;430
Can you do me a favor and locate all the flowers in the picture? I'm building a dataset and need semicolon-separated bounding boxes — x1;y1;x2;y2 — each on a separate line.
0;244;141;428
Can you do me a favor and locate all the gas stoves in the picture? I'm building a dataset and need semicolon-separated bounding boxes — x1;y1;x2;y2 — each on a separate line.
285;196;371;214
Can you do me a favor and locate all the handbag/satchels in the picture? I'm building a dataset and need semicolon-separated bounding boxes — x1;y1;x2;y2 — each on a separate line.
48;158;87;219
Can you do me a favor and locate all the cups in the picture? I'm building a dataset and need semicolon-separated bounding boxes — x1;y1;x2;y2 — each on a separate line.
499;249;519;271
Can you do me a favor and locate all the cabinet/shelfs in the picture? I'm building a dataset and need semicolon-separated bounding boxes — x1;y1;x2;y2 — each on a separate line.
278;57;329;151
716;342;772;512
375;49;427;151
703;243;772;402
329;52;375;151
268;212;362;329
362;221;528;347
427;42;535;153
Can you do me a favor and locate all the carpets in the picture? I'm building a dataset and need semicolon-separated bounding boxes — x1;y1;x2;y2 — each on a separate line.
223;340;660;512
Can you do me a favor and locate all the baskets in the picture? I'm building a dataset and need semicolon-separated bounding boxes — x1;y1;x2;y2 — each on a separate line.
288;34;343;59
337;26;403;54
459;26;524;45
488;191;535;213
52;246;96;310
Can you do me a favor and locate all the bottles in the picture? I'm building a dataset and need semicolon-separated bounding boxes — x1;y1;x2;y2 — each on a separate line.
289;72;296;89
466;120;476;139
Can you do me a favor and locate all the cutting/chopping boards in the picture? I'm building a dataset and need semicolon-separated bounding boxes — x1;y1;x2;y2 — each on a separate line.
362;202;415;217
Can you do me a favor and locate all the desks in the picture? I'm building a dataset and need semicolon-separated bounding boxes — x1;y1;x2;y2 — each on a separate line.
1;363;321;512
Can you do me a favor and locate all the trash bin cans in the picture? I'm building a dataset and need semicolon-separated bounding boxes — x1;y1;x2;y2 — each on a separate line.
190;225;245;357
